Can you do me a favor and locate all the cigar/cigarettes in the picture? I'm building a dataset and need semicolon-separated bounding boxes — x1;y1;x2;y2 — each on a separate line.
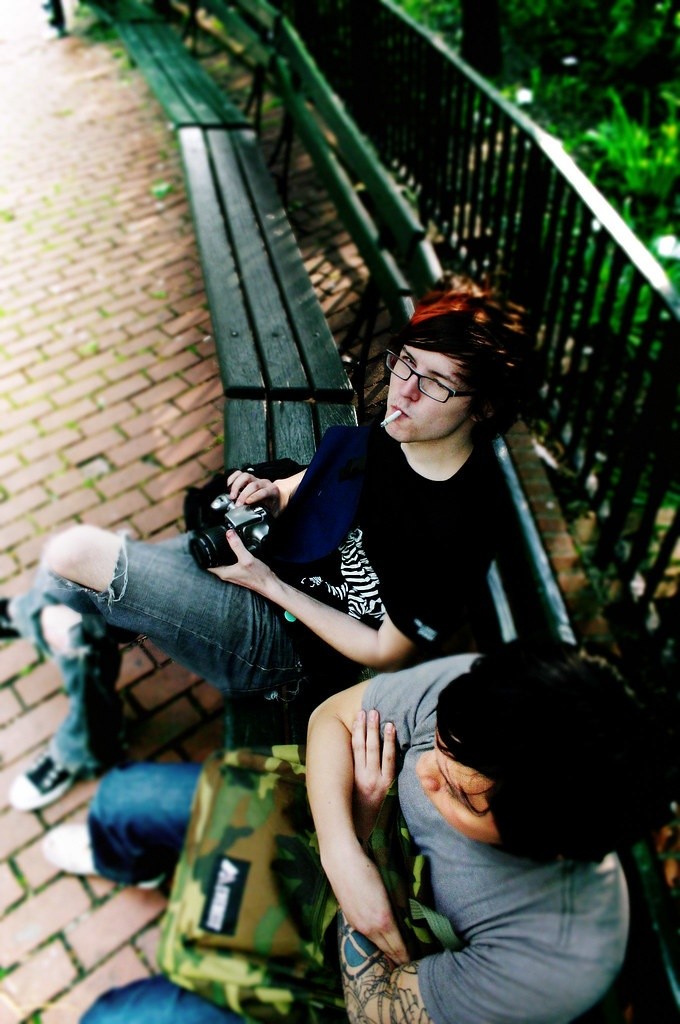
380;409;403;427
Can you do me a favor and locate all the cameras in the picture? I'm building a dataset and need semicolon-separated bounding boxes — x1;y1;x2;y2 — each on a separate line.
189;491;272;569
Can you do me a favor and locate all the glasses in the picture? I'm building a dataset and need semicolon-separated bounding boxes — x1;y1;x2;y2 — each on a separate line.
385;349;471;403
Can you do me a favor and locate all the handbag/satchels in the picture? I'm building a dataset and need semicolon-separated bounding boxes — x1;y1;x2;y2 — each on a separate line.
181;458;310;532
158;746;456;1024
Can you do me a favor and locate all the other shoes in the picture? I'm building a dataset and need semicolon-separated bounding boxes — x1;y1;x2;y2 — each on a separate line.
10;751;75;811
0;598;22;640
44;826;160;891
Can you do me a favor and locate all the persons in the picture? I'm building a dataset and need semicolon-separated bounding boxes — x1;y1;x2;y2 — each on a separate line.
37;633;654;1024
1;283;539;812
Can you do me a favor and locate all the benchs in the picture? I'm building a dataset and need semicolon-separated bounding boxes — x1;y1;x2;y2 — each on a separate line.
179;21;446;398
219;402;680;1023
119;0;279;127
